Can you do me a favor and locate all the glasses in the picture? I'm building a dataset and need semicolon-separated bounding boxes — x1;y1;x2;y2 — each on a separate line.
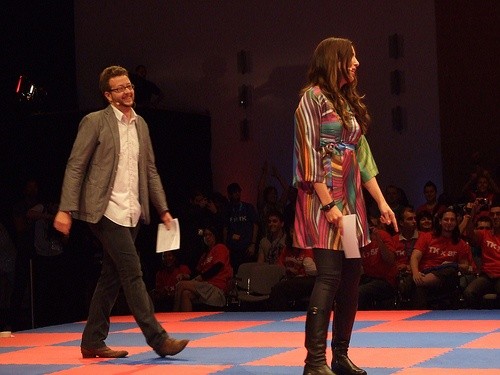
109;84;134;93
477;226;491;230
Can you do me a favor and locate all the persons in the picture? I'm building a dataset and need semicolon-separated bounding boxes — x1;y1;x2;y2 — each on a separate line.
174;164;500;312
148;250;192;312
293;39;398;374
55;66;190;361
0;178;150;332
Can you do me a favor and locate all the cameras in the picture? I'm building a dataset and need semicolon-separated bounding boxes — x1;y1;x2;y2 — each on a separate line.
477;199;487;205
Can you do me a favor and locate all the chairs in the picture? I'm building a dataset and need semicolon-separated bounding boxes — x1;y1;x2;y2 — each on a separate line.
227;262;497;309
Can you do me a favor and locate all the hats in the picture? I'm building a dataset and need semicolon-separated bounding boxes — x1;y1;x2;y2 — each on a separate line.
227;183;241;193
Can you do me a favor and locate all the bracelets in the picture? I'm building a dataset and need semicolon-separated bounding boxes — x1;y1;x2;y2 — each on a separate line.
320;200;334;212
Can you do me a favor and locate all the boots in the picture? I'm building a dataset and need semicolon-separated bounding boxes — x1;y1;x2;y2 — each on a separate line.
302;280;338;375
331;303;368;375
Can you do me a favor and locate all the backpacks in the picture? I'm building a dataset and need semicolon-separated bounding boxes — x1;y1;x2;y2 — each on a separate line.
391;266;417;309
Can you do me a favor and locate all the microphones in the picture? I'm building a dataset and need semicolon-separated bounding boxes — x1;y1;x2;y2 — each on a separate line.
109;102;119;105
349;68;352;70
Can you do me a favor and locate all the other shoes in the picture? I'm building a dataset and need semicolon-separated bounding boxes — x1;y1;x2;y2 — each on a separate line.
156;337;190;358
81;343;129;359
